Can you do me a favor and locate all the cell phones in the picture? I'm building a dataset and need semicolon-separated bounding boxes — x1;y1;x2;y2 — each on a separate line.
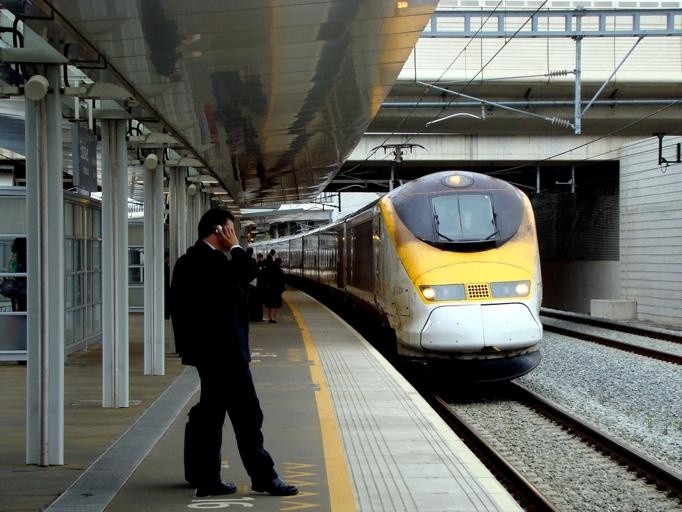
215;223;225;234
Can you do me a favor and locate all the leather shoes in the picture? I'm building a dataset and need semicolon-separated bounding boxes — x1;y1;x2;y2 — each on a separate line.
251;476;298;495
196;480;237;497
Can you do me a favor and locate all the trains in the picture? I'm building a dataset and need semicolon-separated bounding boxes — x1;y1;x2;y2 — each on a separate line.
248;169;548;389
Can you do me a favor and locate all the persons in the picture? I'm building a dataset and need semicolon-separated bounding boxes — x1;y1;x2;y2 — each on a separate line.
170;208;298;499
162;247;287;324
